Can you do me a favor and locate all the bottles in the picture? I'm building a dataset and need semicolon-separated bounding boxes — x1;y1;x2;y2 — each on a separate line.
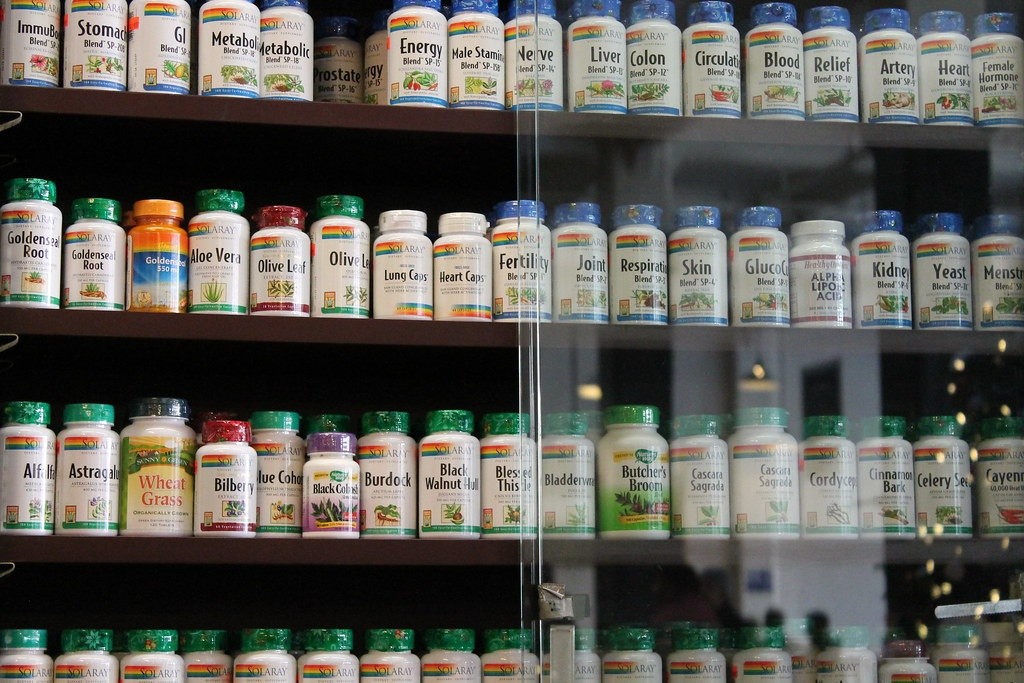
1;1;1024;683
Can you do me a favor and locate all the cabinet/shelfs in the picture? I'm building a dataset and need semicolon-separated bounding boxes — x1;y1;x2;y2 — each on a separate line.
0;85;1024;569
